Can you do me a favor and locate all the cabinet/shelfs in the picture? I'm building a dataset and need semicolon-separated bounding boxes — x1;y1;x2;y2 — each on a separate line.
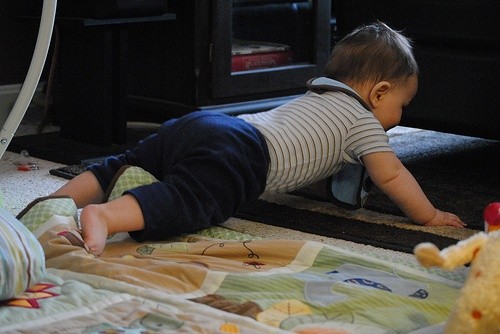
80;0;335;160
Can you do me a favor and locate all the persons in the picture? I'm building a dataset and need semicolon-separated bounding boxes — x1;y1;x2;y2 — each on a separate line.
48;18;470;260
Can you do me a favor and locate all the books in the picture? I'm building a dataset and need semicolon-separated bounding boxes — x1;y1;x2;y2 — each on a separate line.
231;40;291;56
231;53;293;76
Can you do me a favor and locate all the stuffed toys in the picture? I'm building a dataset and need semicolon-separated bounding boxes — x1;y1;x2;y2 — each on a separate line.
413;202;500;334
